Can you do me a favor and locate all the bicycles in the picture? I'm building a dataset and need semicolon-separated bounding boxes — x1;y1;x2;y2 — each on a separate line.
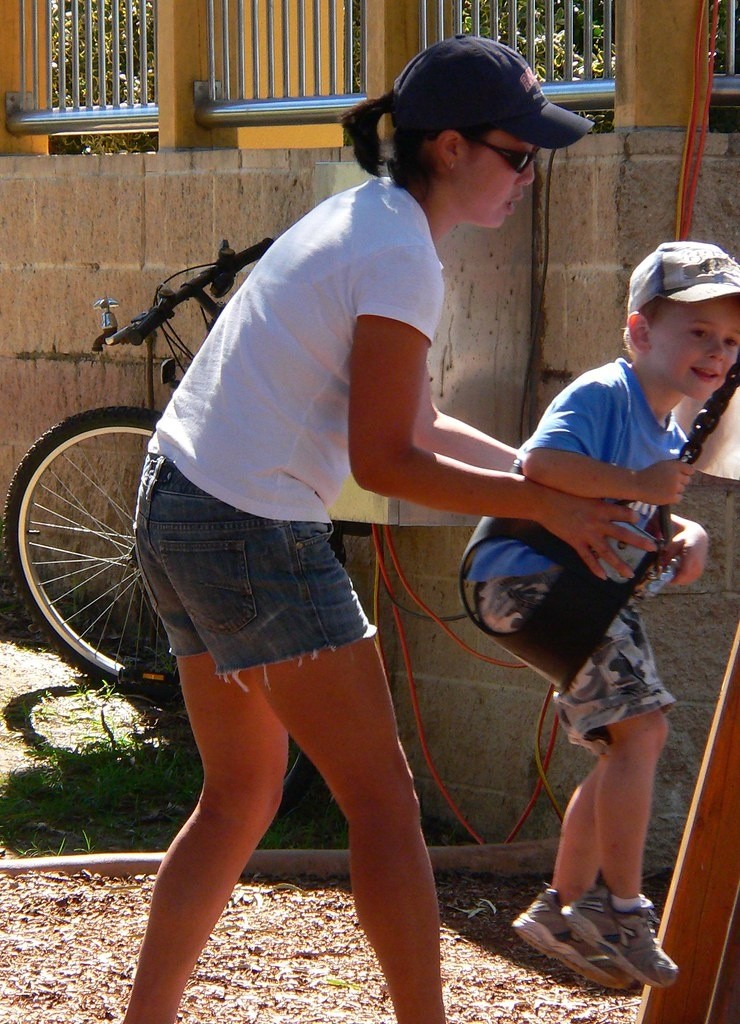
0;233;373;823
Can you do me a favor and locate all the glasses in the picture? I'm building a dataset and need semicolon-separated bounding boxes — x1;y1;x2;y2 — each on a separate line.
457;126;535;175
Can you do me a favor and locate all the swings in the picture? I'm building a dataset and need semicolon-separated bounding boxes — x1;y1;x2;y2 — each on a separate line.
455;351;740;703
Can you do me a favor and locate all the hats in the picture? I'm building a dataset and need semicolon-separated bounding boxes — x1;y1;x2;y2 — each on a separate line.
626;241;740;312
389;32;595;149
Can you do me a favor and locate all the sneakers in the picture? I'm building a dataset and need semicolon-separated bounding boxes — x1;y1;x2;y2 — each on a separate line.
571;884;679;991
513;883;634;991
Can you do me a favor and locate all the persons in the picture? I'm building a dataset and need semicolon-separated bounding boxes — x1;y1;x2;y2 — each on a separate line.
455;239;740;988
121;36;659;1024
